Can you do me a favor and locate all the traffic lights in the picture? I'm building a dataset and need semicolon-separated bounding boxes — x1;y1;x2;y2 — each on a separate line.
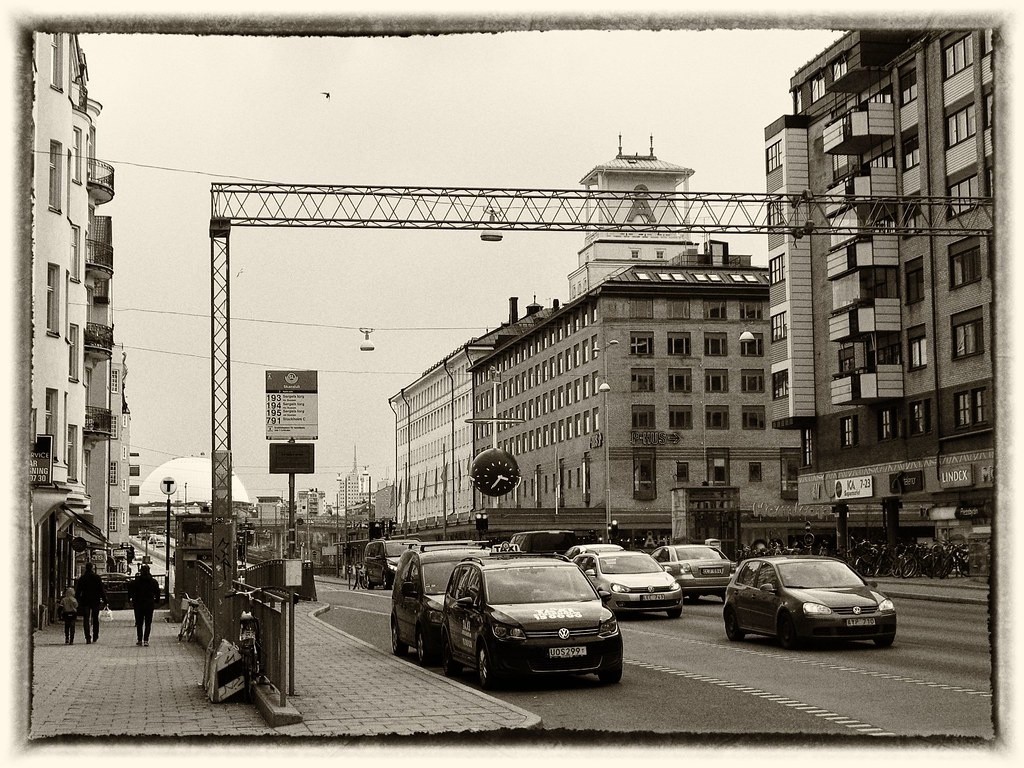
476;512;488;531
389;520;396;533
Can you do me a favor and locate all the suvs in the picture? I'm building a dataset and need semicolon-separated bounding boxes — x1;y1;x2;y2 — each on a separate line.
572;549;682;617
364;538;422;589
440;554;623;690
391;540;492;666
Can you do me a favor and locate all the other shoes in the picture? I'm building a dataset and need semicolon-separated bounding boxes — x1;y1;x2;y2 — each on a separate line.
86;638;91;644
136;640;142;646
144;641;149;646
93;637;97;642
65;638;69;643
69;639;73;645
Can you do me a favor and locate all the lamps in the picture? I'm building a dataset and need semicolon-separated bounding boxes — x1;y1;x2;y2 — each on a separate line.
737;319;755;342
480;206;504;242
598;375;611;393
360;328;375;351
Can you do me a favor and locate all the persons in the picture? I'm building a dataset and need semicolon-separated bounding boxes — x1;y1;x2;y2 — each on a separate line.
74;562;106;644
59;588;77;645
129;565;160;646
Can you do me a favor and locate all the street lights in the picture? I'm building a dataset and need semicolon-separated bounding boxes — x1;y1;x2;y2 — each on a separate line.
592;340;619;523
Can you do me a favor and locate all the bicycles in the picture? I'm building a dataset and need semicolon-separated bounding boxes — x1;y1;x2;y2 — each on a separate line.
736;537;969;578
178;592;205;642
225;585;276;703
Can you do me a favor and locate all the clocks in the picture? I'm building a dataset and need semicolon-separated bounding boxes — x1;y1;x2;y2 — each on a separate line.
483;460;514;492
470;463;481;490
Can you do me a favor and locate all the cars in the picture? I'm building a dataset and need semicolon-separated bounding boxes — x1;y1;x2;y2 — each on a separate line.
509;530;579;553
650;546;736;601
135;553;144;561
149;536;157;544
156;541;165;547
723;554;896;649
133;550;139;556
96;573;165;608
557;544;627;565
